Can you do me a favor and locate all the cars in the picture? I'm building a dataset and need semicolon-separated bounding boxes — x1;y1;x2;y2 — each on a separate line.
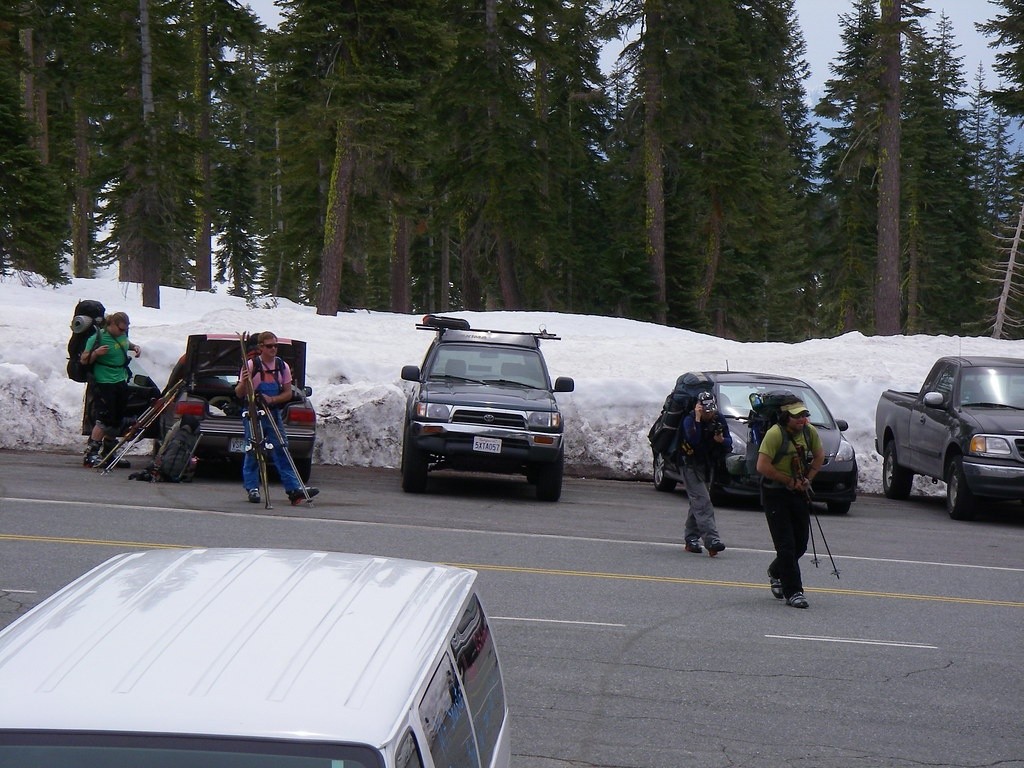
79;332;318;484
652;369;859;515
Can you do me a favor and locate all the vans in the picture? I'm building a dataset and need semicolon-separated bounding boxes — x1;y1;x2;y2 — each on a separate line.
1;542;514;768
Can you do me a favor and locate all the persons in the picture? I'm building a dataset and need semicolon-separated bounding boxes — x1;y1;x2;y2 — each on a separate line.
678;391;732;556
81;312;141;468
756;397;826;609
234;332;320;505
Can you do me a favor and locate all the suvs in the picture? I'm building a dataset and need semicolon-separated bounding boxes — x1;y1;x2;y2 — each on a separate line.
397;313;575;502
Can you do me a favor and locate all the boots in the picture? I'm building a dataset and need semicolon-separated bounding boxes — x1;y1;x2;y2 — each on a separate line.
99;439;131;469
83;437;110;468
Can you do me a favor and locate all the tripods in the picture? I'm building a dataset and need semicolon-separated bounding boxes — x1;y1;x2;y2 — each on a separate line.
246;392;315;508
94;378;187;475
805;492;842;579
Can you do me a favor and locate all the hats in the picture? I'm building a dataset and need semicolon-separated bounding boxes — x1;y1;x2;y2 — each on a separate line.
699;392;717;412
780;395;810;414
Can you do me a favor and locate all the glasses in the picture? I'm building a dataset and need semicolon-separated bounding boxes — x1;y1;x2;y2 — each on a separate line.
790;413;811;419
262;344;278;349
116;323;128;333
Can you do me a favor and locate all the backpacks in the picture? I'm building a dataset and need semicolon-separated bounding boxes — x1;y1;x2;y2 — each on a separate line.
648;373;714;457
67;299;106;382
746;389;799;476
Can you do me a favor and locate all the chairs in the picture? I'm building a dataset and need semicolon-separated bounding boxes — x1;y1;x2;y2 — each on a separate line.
446;359;467;377
502;363;523;377
961;379;990;402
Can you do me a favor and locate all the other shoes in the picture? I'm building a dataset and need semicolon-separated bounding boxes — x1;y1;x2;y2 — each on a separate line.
247;487;261;503
288;485;320;503
785;593;808;609
685;539;703;553
707;542;725;557
768;569;782;597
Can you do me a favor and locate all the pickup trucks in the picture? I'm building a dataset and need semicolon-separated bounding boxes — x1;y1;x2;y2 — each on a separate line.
874;355;1024;522
